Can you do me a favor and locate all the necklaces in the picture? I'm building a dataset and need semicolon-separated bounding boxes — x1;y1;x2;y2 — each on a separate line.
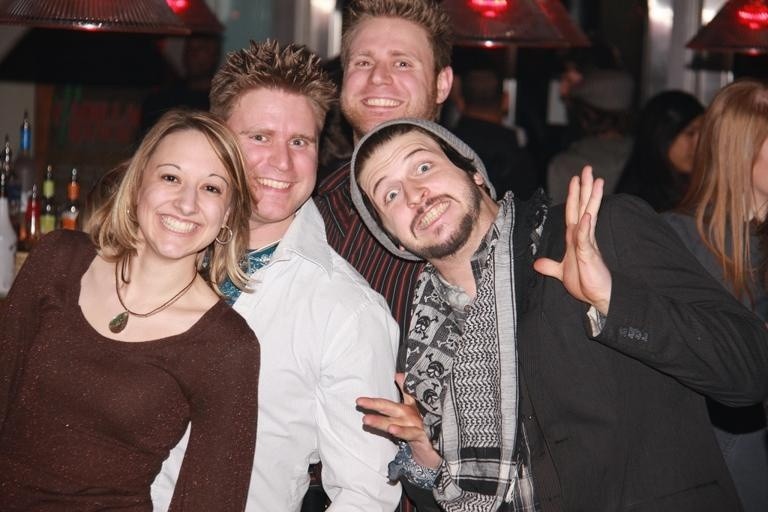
106;255;200;332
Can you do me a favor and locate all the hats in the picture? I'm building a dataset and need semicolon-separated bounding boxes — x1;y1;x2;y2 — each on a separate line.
567;71;635;112
349;118;497;262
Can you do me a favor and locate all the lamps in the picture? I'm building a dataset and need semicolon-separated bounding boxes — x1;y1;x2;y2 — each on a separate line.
733;1;767;32
466;0;509;18
167;0;192;15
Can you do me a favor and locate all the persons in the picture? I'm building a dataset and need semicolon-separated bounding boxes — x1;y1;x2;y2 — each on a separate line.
300;0;454;512
73;159;133;237
316;56;766;512
349;115;767;511
151;39;405;512
0;110;260;511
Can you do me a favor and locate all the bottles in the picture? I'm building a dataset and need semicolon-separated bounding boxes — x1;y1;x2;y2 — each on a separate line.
0;168;17;296
25;184;39;248
39;162;55;232
0;133;13;192
14;109;33;249
59;166;79;231
